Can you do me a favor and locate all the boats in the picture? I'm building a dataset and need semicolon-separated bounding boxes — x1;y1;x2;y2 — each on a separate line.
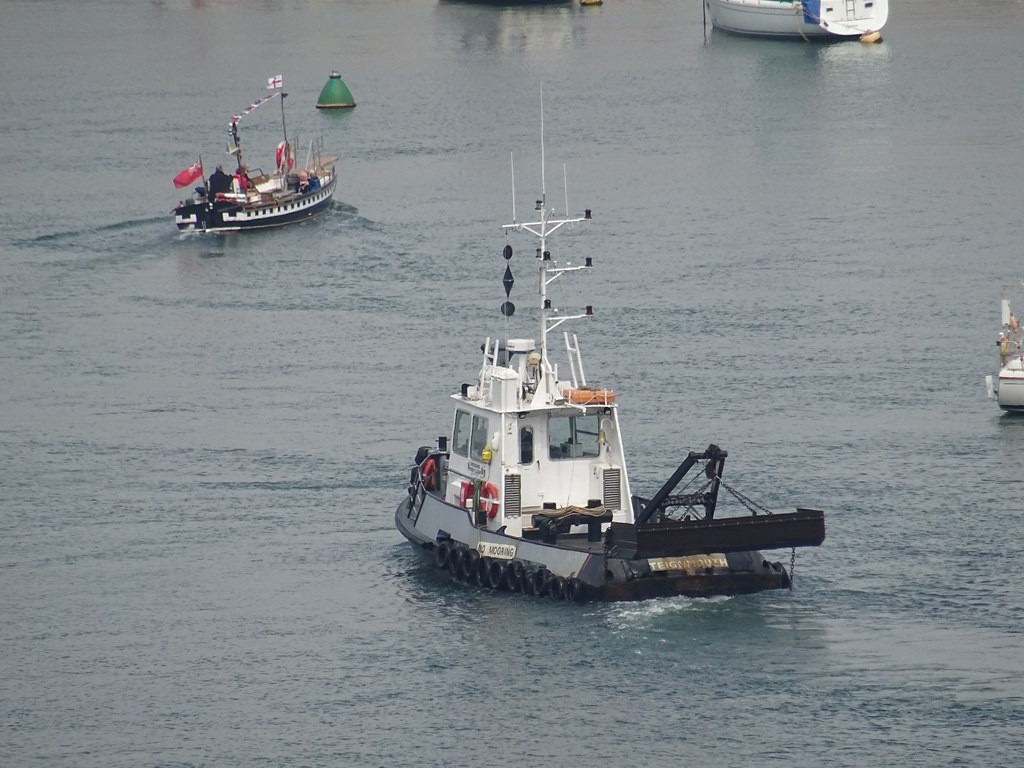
981;296;1024;414
394;80;827;605
167;73;340;244
703;0;892;47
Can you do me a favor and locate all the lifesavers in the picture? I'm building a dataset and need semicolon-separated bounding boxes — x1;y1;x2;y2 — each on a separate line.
276;141;295;173
422;459;437;491
478;483;499;521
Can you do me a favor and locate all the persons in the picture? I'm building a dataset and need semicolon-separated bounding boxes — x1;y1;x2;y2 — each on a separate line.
294;171;317;194
234;167;248;194
208;163;233;201
309;170;322;190
